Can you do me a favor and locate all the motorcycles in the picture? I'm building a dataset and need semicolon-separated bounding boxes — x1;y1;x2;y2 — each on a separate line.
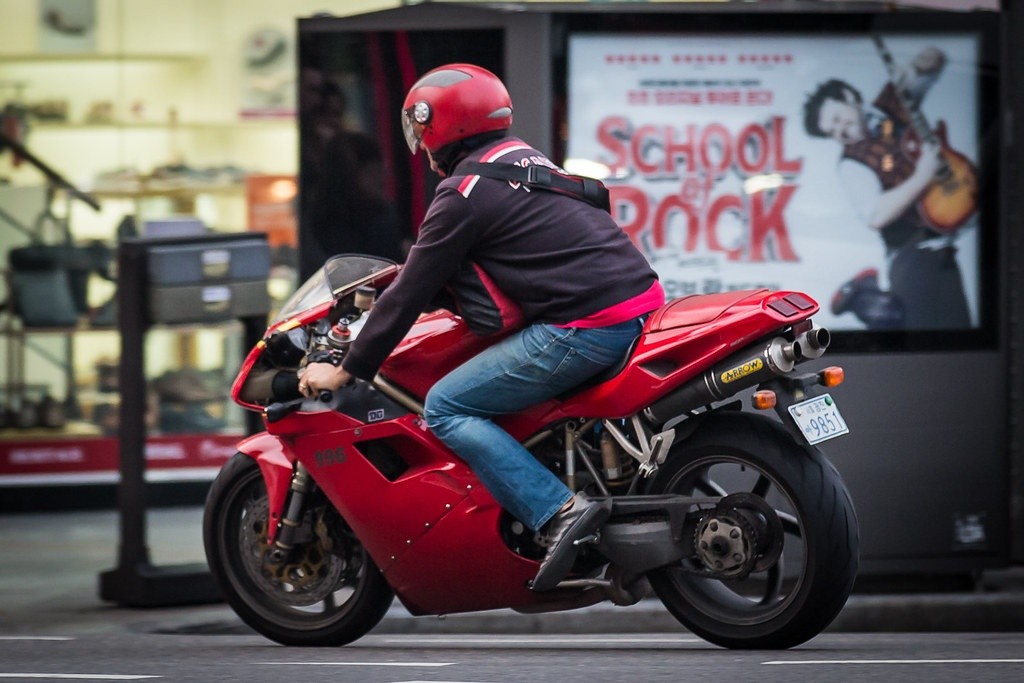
202;252;861;651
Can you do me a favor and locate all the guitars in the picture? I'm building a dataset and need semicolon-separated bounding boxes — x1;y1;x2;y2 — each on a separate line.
874;33;978;232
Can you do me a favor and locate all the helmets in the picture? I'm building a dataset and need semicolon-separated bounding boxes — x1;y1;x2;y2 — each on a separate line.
403;63;512;155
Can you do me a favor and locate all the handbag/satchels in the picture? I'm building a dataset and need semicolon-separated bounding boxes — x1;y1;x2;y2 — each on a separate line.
443;158;613;340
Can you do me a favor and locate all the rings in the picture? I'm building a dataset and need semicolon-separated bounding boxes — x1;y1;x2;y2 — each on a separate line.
299;383;307;388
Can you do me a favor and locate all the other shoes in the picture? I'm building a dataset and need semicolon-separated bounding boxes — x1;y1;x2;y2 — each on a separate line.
831;268;879;315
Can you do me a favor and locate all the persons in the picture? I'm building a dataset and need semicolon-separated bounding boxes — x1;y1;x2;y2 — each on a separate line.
298;64;665;590
804;47;973;329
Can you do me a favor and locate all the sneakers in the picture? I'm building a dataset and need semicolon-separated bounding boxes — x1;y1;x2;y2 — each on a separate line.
529;490;609;592
